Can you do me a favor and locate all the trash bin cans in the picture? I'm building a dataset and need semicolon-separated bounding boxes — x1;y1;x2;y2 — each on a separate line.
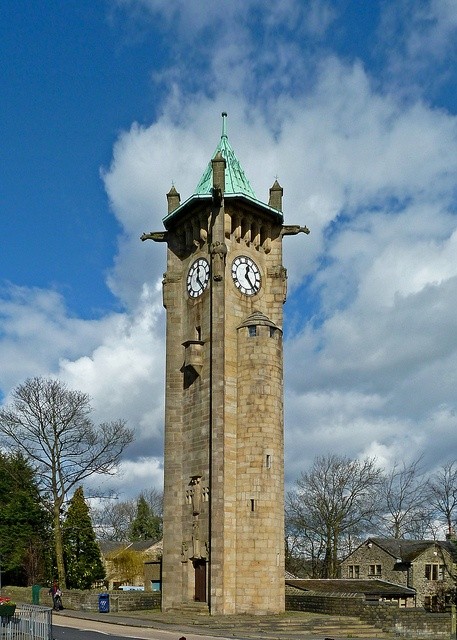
99;594;109;612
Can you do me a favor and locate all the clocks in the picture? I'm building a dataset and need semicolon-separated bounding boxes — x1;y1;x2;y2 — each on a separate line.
186;257;209;300
231;255;262;296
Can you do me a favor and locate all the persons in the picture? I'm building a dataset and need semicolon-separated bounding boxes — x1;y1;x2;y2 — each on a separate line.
49;583;63;611
